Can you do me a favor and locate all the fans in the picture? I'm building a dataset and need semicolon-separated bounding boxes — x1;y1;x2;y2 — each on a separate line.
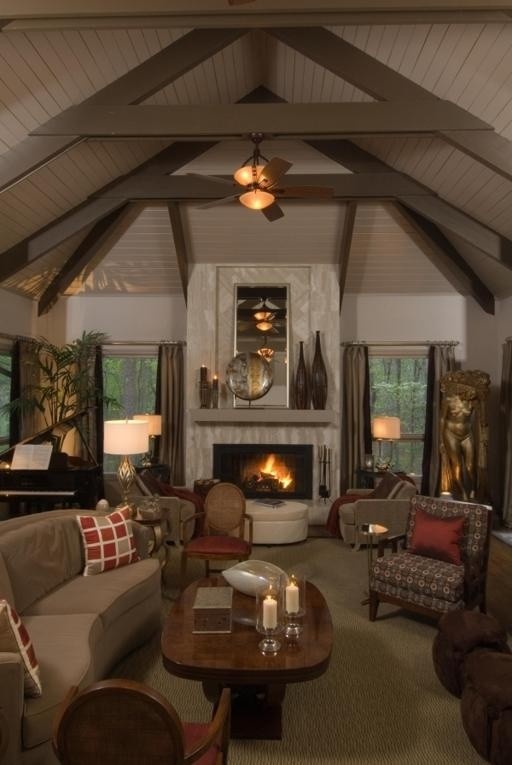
186;132;335;223
238;297;284;343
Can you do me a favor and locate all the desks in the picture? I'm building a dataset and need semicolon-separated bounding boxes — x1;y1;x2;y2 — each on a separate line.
1;472;80;515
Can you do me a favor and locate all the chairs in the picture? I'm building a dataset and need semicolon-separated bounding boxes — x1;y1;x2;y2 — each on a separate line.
368;495;493;621
121;468;201;546
182;483;252;577
50;681;232;765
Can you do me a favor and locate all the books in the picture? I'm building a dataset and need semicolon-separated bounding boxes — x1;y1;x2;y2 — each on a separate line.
254;498;286;510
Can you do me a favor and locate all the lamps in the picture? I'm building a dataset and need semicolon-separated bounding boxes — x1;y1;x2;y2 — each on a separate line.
253;310;272;333
257;337;274;363
232;133;275;210
103;419;150;506
372;416;400;471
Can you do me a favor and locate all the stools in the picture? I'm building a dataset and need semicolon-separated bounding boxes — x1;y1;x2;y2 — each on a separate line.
459;649;512;765
436;612;508;700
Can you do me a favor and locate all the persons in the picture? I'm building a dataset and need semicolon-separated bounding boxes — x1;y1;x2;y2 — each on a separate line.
438;369;491;500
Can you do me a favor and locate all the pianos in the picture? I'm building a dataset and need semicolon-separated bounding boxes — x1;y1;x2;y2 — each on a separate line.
0;408;102;517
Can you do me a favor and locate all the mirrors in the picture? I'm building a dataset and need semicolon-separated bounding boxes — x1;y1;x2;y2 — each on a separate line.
233;284;291;411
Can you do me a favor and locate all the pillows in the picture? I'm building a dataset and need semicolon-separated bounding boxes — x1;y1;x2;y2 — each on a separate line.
134;469;160;497
410;511;467;566
1;599;43;699
77;506;141;577
364;474;404;498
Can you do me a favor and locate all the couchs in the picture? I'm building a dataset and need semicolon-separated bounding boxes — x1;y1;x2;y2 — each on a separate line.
235;499;309;546
337;472;417;551
1;509;164;764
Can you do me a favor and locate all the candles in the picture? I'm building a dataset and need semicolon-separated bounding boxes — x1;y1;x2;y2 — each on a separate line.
263;585;278;631
213;375;218;408
200;365;208;407
285;574;299;614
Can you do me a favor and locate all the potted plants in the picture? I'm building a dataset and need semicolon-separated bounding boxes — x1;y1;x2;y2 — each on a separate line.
1;328;126;497
